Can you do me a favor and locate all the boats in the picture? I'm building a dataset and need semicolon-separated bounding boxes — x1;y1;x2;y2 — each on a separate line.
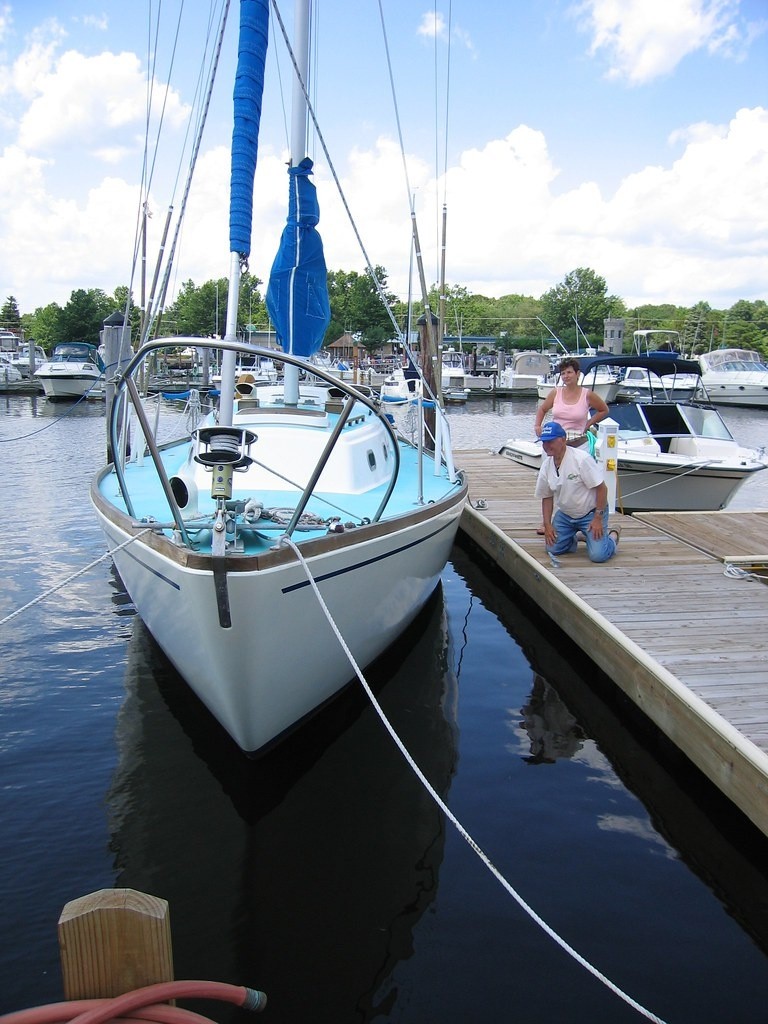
139;345;424;405
103;608;460;1024
690;348;768;408
536;348;624;405
32;343;106;402
618;329;700;406
0;346;23;384
498;356;767;512
0;328;48;378
439;347;470;405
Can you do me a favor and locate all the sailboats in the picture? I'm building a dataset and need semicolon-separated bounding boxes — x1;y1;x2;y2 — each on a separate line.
84;2;470;766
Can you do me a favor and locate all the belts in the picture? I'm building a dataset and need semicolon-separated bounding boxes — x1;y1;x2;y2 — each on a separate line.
564;508;597;520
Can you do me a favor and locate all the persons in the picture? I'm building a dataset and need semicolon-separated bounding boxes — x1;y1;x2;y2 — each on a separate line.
534;421;622;562
535;361;610;534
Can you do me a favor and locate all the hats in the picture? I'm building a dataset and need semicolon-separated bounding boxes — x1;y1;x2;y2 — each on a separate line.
533;421;566;443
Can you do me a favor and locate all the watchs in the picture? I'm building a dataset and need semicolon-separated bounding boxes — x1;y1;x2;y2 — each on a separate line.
596;509;604;515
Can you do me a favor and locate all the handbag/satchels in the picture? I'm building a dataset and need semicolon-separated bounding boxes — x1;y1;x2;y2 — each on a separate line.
584;428;597;457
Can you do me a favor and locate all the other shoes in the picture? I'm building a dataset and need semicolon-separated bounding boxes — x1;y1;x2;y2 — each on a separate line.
536;523;545;535
608;524;622;546
